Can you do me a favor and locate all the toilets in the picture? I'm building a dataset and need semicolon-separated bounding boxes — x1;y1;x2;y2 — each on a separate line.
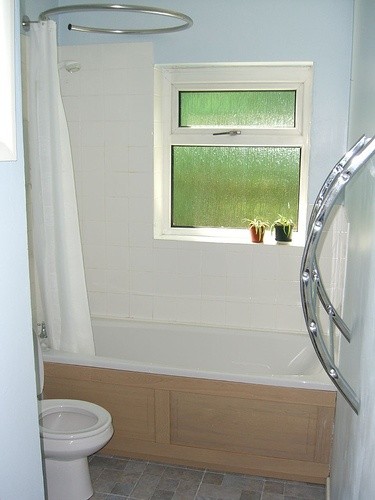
33;330;114;499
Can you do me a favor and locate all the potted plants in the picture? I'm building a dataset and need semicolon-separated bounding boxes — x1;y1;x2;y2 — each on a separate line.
241;217;269;242
271;215;296;241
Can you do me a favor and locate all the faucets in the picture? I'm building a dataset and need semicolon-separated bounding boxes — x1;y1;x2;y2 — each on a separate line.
36;322;47;339
